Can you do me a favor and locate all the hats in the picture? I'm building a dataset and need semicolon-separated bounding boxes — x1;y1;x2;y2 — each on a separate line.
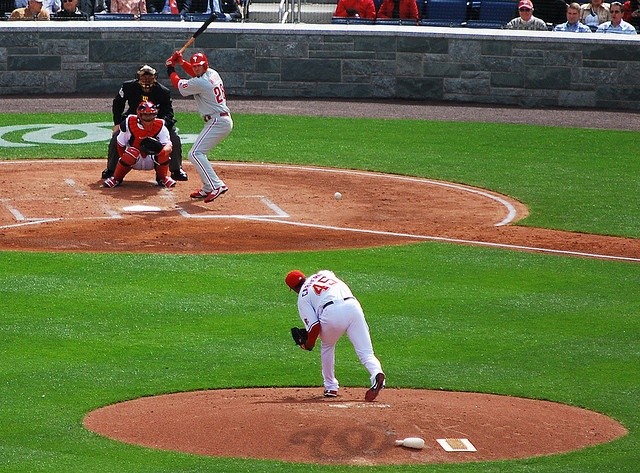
518;0;533;10
285;271;306;292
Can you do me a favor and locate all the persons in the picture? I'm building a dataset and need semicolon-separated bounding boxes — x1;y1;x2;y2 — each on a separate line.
166;50;234;203
552;2;592;32
377;0;419;26
15;0;61;15
61;0;108;18
146;0;187;14
102;65;188;181
579;0;611;32
622;0;640;26
179;0;242;22
596;1;637;35
333;0;377;19
51;0;89;20
506;0;548;30
285;269;386;402
9;0;49;21
110;0;148;18
104;100;176;188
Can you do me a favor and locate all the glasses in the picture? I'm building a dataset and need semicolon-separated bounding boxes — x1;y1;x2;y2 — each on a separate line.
610;10;622;14
63;0;72;3
519;9;531;12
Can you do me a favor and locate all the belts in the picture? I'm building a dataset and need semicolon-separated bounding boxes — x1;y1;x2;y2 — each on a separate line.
204;113;232;123
321;296;355;314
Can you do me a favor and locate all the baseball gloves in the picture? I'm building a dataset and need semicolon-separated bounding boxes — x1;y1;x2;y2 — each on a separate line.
140;137;163;156
291;327;312;351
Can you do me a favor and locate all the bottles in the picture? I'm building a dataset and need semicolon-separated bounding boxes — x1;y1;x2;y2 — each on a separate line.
394;436;425;449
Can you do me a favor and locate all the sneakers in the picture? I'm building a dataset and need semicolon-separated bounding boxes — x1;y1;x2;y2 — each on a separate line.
189;189;208;198
364;373;384;402
102;167;112;179
204;185;228;203
323;389;337;397
157;176;176;188
103;176;119;188
171;168;188;181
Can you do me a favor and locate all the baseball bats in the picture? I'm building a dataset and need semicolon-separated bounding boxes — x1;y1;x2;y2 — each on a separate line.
172;13;217;60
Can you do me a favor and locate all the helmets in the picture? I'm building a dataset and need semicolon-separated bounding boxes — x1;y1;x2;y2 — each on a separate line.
190;52;208;72
137;64;158;94
137;99;157;131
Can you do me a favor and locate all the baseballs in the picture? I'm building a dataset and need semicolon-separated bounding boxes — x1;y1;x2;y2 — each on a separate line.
334;192;341;200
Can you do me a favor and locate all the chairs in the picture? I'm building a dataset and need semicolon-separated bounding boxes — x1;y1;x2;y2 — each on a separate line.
477;0;520;23
425;0;472;25
